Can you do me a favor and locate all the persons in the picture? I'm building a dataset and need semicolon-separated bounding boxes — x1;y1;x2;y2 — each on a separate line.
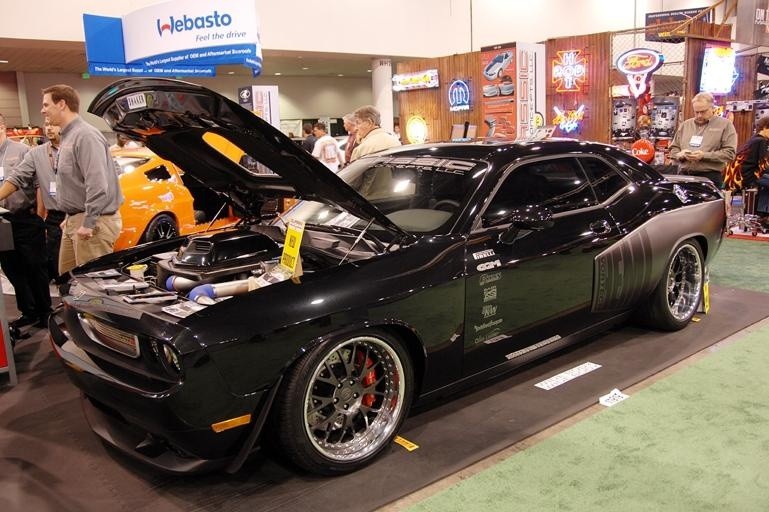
349;106;403;164
39;85;123;280
106;129;128;149
0;116;68;303
0;113;56;329
665;92;740;192
300;123;319;154
341;114;360;165
393;124;401;140
727;116;769;232
310;122;345;174
124;136;145;149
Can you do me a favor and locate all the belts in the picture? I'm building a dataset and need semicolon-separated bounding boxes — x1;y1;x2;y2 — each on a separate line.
98;210;119;216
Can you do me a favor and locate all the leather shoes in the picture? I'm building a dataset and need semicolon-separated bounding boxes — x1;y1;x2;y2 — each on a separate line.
9;310;53;328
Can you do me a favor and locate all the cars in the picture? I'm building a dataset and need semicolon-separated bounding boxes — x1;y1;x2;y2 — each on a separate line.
110;111;300;253
47;77;733;488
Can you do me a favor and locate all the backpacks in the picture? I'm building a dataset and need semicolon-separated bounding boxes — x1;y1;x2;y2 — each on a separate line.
724;145;753;193
